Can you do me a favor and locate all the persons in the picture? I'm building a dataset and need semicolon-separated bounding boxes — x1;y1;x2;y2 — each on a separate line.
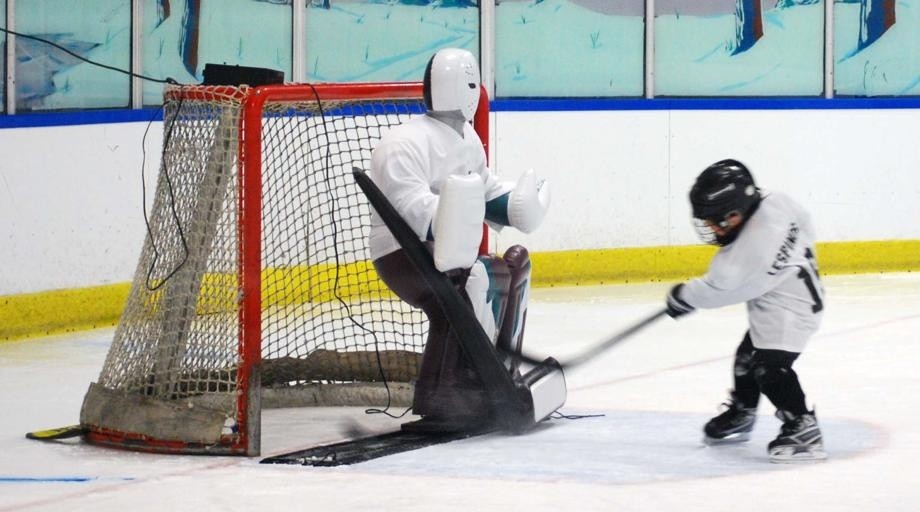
368;46;567;426
663;159;829;458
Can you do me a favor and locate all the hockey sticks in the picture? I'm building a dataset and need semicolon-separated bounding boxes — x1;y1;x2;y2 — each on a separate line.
495;313;666;371
350;166;565;432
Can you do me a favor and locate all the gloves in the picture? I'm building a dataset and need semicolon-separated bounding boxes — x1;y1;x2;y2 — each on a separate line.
665;284;693;319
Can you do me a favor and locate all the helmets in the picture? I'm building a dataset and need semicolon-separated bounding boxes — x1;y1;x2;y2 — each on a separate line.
423;47;480;123
689;158;761;246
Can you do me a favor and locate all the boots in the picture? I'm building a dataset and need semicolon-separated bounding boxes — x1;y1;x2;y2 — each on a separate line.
767;406;822;454
702;392;757;438
